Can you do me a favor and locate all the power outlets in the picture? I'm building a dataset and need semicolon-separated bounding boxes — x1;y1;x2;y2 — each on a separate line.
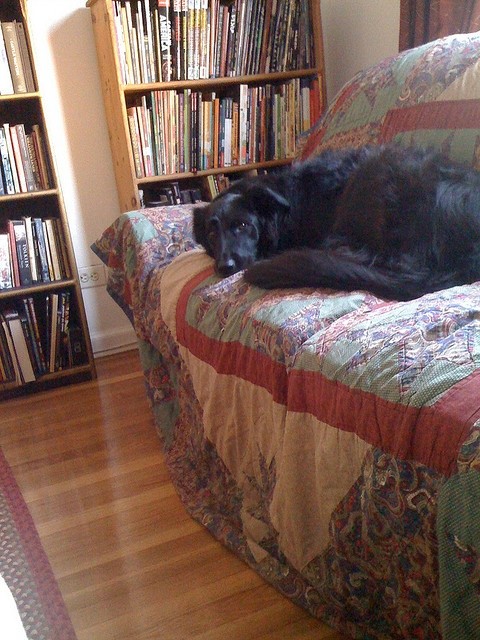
78;265;108;290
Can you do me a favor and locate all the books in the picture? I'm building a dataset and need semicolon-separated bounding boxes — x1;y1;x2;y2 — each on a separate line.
127;78;323;177
0;215;71;291
0;292;89;388
114;0;315;85
0;123;52;196
138;163;290;207
0;21;36;96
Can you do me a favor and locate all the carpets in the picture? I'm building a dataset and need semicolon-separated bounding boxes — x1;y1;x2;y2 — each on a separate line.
2;446;81;640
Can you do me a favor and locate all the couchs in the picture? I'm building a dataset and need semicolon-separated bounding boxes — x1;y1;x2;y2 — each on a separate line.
92;32;480;639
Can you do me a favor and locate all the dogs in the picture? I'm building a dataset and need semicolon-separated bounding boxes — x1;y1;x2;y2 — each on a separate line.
192;137;480;302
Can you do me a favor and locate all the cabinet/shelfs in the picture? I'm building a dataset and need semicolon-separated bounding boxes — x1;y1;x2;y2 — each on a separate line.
0;0;97;399
86;0;329;214
